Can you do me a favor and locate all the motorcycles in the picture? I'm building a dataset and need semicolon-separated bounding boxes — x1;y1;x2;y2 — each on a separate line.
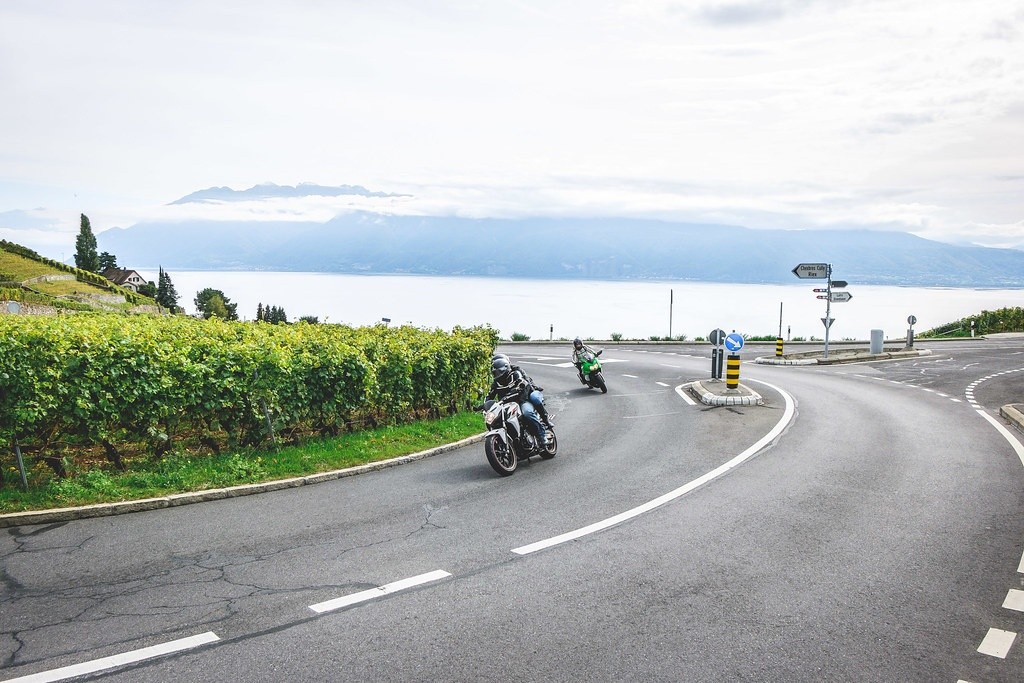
473;382;558;475
569;348;608;394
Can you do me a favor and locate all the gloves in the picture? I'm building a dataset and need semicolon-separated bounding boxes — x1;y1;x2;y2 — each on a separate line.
509;386;518;394
478;404;484;410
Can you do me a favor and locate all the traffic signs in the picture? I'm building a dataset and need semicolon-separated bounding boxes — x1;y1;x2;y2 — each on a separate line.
791;263;829;280
831;280;849;288
829;291;853;302
813;289;828;293
817;296;828;299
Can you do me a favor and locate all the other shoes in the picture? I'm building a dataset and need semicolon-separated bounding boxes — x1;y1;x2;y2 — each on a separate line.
537;434;549;447
541;414;554;429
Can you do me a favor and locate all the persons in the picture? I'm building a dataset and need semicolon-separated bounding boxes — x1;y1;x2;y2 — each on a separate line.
571;339;598;385
483;353;555;446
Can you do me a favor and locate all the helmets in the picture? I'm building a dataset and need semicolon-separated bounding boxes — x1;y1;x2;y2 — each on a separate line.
491;359;514;379
574;338;582;350
491;354;510;364
